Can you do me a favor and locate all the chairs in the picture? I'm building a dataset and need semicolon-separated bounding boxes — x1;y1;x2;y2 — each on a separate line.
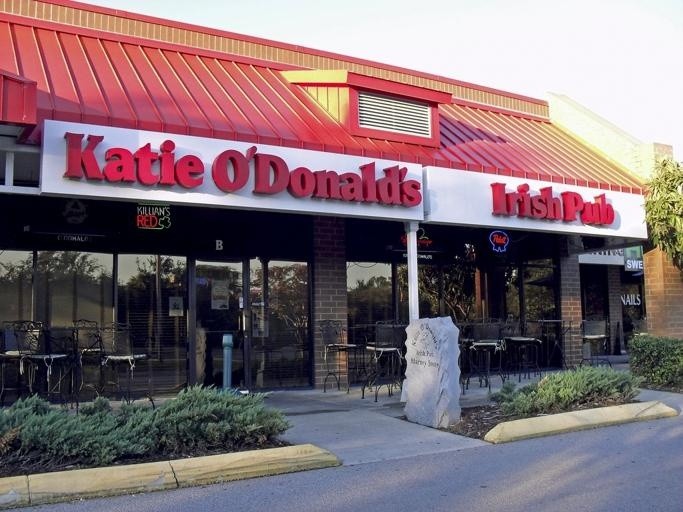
271;333;308;388
0;319;156;411
319;315;612;402
